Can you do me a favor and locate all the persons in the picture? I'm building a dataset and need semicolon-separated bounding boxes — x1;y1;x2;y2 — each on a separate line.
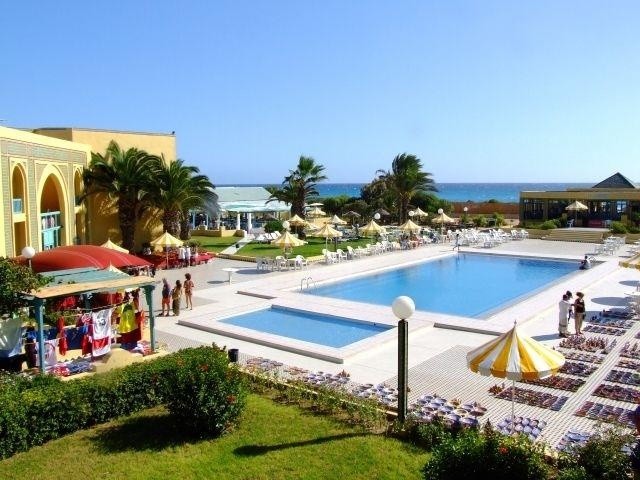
125;244;199;317
452;233;461;251
355;223;360;237
579;255;591;269
400;227;436;250
558;291;586;338
25;326;37;369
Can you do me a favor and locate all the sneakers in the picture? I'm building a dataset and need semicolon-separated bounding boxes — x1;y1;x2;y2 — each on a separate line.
158;313;170;317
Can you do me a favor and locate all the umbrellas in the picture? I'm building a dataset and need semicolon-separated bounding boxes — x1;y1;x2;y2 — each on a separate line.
414;206;428;221
288;206;347;261
360;218;386;243
431;212;454;235
565;199;589;226
465;318;565;436
150;230;183;270
397;219;422;232
99;237;130;255
270;229;307;259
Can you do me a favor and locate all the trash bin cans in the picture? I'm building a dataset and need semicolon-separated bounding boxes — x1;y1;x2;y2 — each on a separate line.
228;349;238;362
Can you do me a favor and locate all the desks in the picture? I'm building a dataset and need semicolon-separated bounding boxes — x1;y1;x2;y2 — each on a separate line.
222;267;239;284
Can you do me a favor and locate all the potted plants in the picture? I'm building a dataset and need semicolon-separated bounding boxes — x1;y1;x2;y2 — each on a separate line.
183;239;202;257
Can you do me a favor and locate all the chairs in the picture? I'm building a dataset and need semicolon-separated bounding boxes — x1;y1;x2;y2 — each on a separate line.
566;218;575;228
255;227;529;273
584;233;640;257
